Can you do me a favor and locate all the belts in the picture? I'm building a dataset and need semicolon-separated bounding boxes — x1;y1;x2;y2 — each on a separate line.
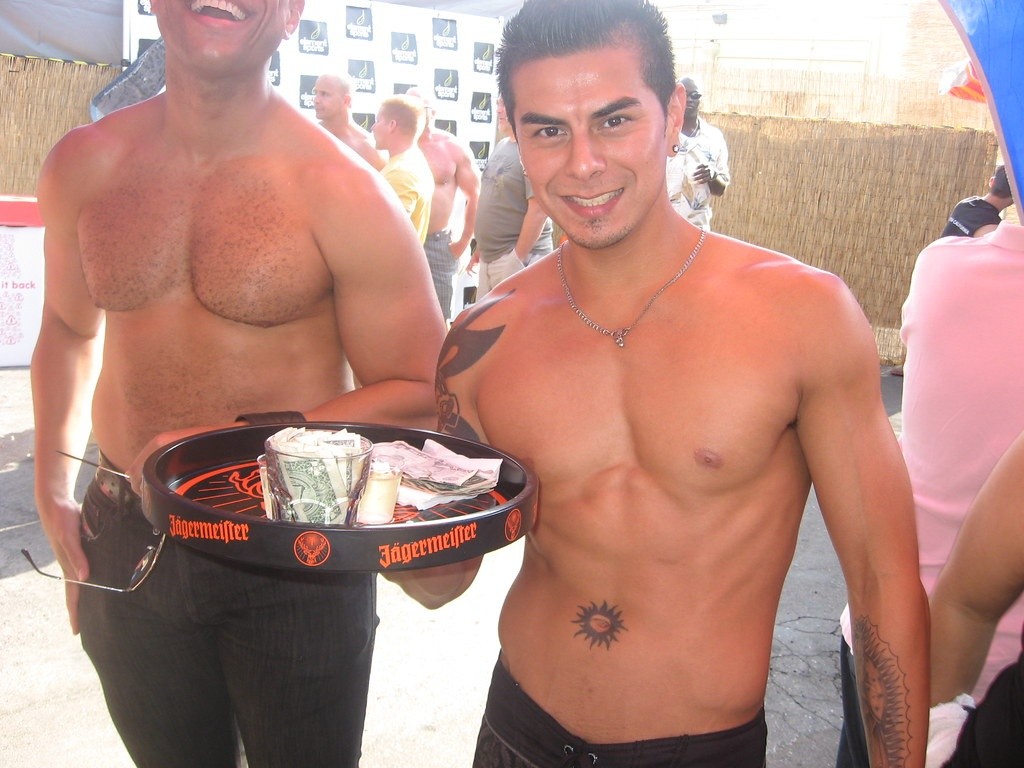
91;449;131;509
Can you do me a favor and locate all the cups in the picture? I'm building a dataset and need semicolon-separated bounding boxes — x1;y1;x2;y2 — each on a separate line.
356;455;406;524
257;453;272;520
266;429;373;522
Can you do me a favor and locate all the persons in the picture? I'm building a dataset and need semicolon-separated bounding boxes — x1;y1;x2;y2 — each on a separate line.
379;0;931;768
310;66;730;333
834;166;1024;768
29;0;449;767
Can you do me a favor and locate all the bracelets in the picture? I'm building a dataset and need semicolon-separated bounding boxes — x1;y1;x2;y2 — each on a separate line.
708;171;718;183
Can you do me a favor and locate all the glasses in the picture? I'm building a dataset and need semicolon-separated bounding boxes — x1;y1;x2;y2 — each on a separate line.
20;448;168;595
687;90;703;100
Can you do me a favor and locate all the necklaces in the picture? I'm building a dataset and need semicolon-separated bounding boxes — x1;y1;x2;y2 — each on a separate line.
689;118;698;136
558;226;706;348
